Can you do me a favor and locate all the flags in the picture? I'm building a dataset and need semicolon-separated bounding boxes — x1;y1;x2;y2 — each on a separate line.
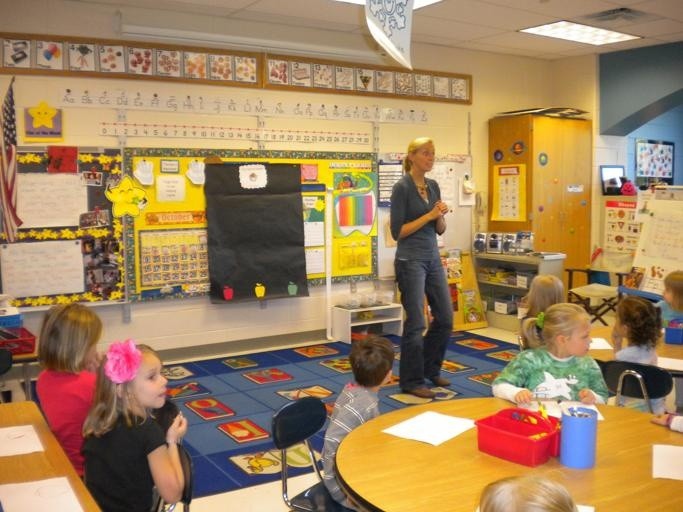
0;84;23;245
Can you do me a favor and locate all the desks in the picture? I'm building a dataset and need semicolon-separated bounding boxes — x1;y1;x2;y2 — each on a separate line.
585;325;683;376
336;395;683;512
1;338;45;401
0;399;104;512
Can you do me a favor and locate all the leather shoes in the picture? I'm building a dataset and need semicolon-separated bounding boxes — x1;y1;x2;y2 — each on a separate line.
402;385;436;397
428;376;451;386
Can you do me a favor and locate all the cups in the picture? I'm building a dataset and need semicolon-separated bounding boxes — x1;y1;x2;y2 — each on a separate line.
560;407;597;468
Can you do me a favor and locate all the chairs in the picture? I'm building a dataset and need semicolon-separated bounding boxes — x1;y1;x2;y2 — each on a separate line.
272;397;353;512
154;445;194;512
603;361;672;411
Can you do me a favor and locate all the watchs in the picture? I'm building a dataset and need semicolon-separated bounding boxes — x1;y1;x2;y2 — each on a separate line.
166;437;183;448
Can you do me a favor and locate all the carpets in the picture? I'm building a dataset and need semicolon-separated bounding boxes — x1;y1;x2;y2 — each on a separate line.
156;327;534;498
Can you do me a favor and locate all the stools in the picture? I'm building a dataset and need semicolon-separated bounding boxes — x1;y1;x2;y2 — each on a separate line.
570;283;622;324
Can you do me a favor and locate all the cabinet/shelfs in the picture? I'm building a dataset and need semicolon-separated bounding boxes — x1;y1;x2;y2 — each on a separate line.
332;303;404;344
489;115;592;306
474;250;566;328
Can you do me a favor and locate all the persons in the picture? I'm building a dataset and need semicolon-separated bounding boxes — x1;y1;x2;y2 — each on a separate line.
36;303;103;483
652;270;683;321
389;137;453;399
320;337;394;512
479;475;579;512
492;303;609;406
518;275;564;352
80;343;187;512
607;295;667;418
